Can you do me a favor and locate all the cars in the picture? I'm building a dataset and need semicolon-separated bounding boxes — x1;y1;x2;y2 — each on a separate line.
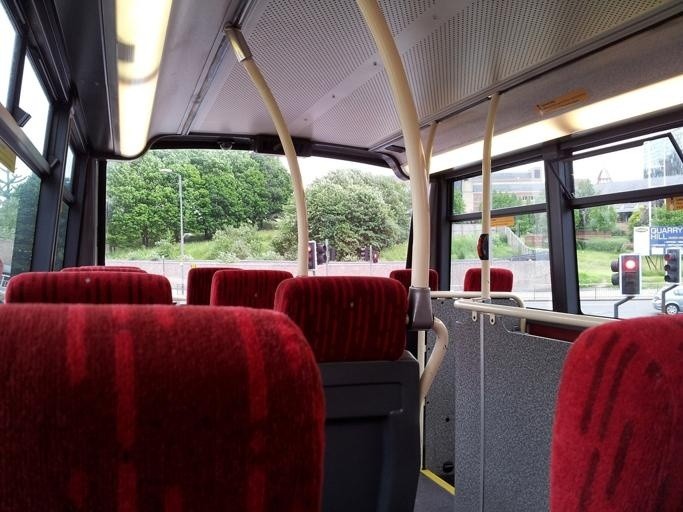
654;284;683;315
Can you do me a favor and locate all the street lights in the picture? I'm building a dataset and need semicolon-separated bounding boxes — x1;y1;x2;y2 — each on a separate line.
160;168;185;287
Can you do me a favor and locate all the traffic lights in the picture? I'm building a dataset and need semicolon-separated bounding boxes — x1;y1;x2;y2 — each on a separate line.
308;241;317;270
373;253;378;263
317;243;327;263
664;248;680;282
611;255;621;295
361;247;369;261
623;255;640;294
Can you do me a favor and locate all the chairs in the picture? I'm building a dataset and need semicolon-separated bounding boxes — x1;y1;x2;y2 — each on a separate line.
209;266;293;310
271;273;424;509
463;265;517;298
546;313;678;510
388;268;439;292
2;298;331;512
4;270;174;303
184;267;244;303
